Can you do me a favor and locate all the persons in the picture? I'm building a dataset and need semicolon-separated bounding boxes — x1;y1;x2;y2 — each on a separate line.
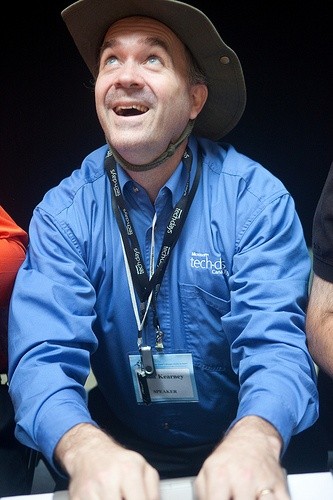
0;0;319;500
305;161;333;380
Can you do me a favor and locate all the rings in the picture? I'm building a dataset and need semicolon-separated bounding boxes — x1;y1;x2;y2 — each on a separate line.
257;487;274;499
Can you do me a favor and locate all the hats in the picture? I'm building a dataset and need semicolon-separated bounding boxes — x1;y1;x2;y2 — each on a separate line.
59;0;247;143
